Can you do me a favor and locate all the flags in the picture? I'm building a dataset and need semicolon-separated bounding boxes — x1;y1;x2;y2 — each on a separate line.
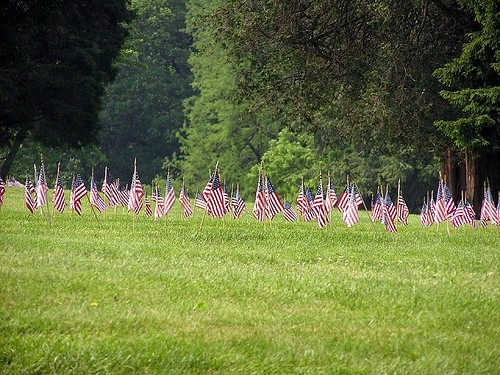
253;173;267;222
33;173;43;209
283;198;298;222
113;178;129;207
122;170;177;220
201;171;228;219
73;172;88;216
0;177;5;208
207;169;246;220
179;183;192;218
296;179;500;234
102;168;115;207
90;176;108;213
6;175;17;187
265;175;284;222
25;176;37;214
70;177;74;209
37;168;46;208
52;171;66;214
194;192;206;210
